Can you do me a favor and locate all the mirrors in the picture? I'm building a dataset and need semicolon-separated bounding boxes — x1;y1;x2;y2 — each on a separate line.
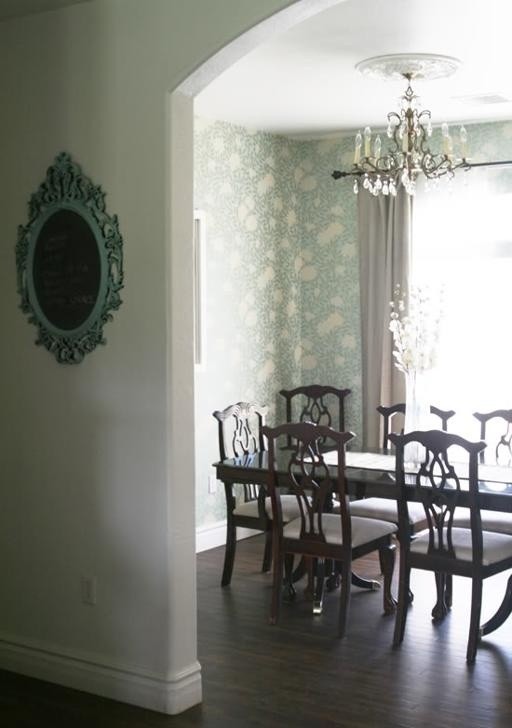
12;152;126;369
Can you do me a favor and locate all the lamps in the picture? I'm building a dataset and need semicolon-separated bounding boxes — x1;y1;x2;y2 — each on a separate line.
349;51;475;202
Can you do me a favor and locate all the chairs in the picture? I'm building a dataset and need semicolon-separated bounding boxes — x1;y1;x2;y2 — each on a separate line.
330;402;456;605
213;400;341;598
440;407;512;609
278;384;353;452
384;429;511;667
259;421;397;640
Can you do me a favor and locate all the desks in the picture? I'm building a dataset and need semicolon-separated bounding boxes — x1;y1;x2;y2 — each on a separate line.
211;455;512;637
